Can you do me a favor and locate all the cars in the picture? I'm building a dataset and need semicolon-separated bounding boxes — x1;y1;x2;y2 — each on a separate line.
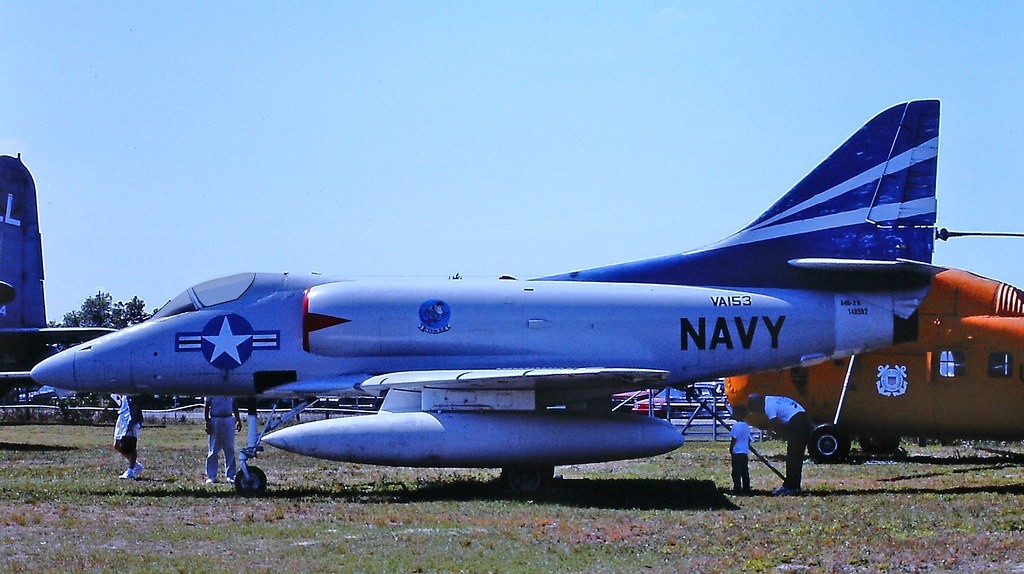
613;381;729;416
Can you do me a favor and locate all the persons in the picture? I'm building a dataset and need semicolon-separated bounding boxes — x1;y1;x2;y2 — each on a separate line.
729;405;751;496
748;394;810;495
113;393;144;479
205;395;242;483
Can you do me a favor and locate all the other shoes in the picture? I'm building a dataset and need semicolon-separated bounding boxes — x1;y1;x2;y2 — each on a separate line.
119;468;134;478
226;475;236;483
772;486;801;495
132;461;144;477
206;477;216;484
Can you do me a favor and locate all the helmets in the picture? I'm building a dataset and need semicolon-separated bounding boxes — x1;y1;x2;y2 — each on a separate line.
747;393;764;411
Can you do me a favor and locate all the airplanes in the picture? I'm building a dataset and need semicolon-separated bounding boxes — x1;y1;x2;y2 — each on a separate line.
27;98;1024;495
